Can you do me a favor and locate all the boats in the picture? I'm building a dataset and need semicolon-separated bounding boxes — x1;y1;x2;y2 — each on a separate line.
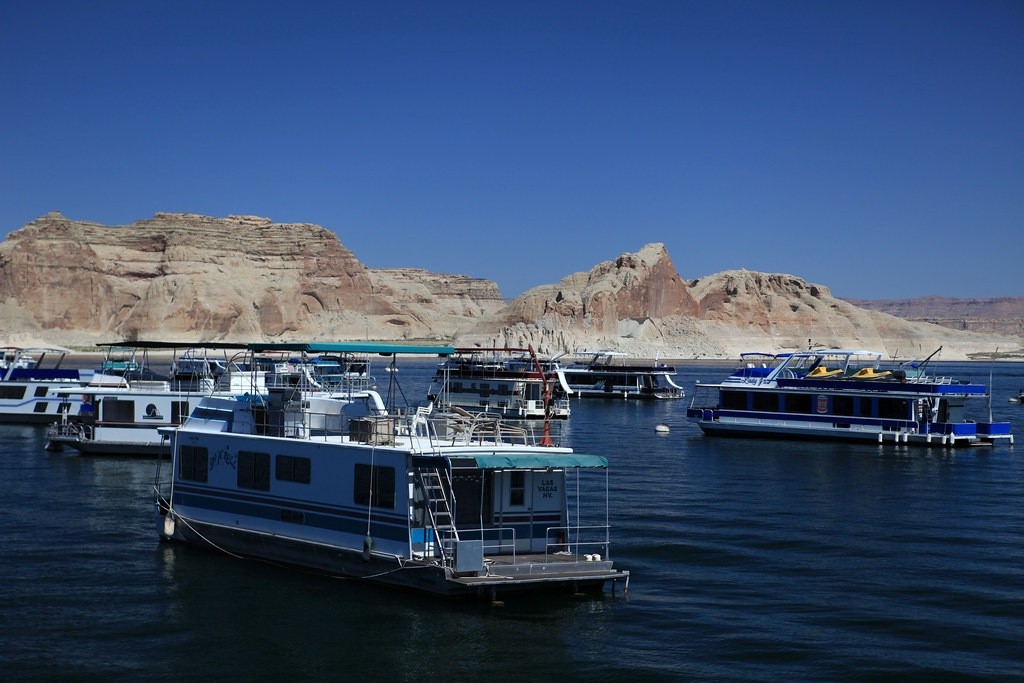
687;345;1014;450
423;341;685;401
0;332;630;607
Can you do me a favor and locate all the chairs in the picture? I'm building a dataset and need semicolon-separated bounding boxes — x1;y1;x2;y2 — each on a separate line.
405;401;528;447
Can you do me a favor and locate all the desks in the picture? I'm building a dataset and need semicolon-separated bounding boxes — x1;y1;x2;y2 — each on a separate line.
369;414;407;436
